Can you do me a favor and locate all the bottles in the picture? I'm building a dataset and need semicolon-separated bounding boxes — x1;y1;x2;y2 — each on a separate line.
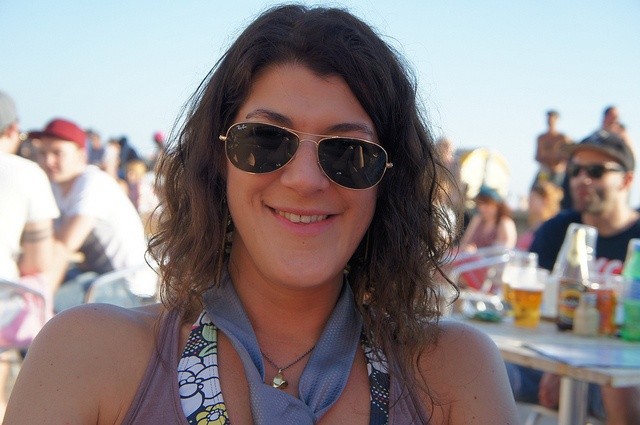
557;229;589;331
623;239;640;341
572;292;600;336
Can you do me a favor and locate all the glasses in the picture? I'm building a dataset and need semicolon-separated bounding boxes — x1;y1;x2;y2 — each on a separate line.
567;160;626;177
219;121;393;191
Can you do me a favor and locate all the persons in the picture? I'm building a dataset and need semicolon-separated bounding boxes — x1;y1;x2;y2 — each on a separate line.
502;131;640;424
517;180;564;251
19;119;160;309
3;5;520;425
432;186;518;293
2;94;70;351
123;155;168;219
602;106;627;143
535;111;575;183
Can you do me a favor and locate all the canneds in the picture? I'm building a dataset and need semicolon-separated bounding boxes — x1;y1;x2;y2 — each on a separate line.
587;282;617;334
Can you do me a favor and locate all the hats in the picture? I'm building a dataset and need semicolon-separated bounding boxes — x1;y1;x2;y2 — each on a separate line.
28;120;86;148
568;130;635;170
471;185;500;203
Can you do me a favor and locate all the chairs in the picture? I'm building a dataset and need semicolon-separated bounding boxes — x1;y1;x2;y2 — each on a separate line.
84;251;166;309
449;249;538;307
0;279;52;356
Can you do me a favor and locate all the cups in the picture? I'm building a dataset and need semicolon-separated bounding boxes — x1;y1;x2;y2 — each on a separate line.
502;251;538;319
508;285;543;329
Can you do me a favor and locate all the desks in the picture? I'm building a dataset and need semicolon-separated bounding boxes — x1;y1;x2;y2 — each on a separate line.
422;308;640;425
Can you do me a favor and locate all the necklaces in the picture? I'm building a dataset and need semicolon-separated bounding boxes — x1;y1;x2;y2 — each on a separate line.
259;347;314;389
